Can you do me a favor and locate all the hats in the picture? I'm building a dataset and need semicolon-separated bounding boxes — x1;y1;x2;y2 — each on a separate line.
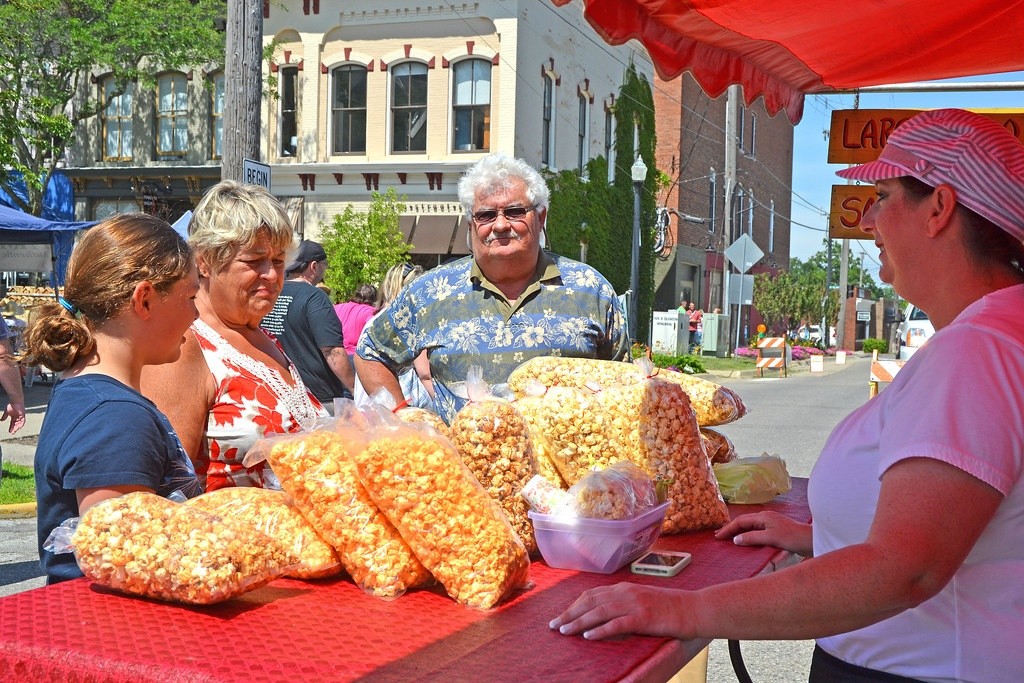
836;108;1024;246
286;240;325;272
0;301;24;317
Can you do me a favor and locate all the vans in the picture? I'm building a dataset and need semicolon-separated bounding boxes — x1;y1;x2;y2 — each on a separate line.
884;303;935;361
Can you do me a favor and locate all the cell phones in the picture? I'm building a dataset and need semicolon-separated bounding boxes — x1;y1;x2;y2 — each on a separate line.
631;550;691;577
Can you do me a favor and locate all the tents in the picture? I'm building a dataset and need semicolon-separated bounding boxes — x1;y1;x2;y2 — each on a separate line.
0;205;101;304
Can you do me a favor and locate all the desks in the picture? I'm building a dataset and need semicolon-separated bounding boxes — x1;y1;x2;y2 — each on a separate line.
0;475;812;683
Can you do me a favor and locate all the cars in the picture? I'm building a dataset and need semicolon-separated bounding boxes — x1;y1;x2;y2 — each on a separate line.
797;325;837;347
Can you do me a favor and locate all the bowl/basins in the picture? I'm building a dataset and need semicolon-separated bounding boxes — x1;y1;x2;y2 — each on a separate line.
527;497;673;574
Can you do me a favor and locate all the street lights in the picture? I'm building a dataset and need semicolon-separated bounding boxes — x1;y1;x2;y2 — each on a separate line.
627;154;648;347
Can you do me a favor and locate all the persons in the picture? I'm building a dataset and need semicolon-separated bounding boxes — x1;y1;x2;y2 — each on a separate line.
24;211;205;584
317;263;427;399
141;180;330;494
260;239;355;417
0;313;26;435
677;300;721;353
549;106;1024;683
352;153;630;412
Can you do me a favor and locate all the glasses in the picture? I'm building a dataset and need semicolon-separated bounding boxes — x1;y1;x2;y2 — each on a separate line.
469;203;538;223
403;264;414;280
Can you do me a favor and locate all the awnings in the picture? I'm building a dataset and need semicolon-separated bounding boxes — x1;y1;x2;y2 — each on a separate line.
552;0;1024;125
